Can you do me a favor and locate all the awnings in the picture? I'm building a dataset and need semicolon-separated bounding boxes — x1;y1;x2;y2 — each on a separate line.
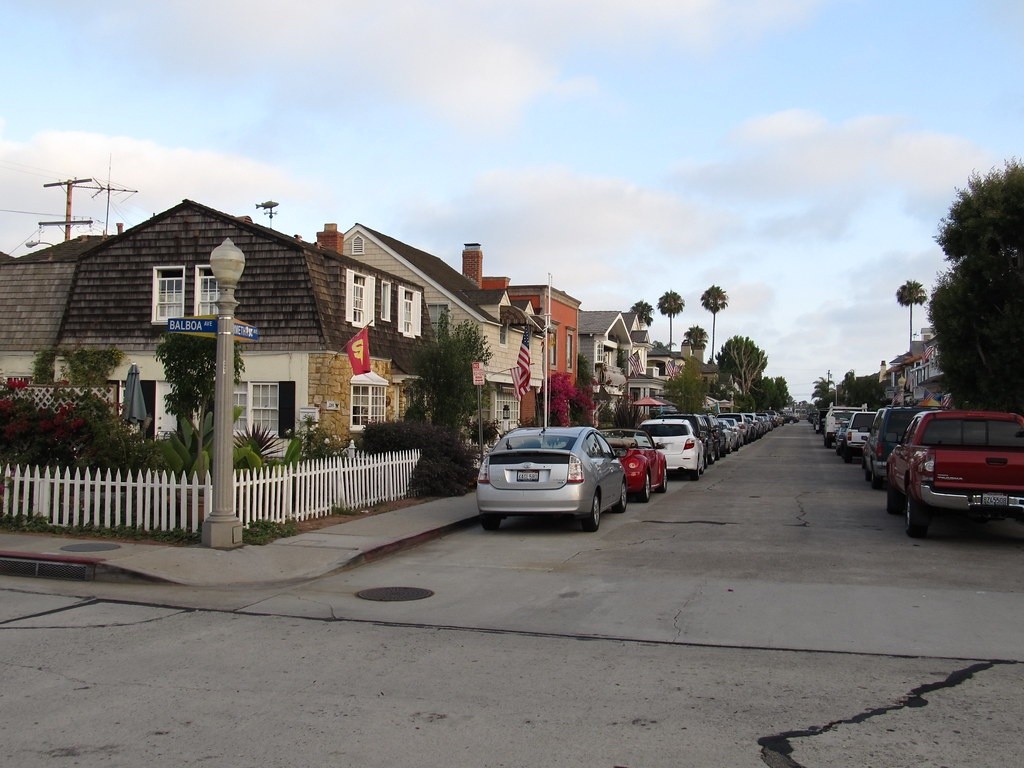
635;397;671;406
704;397;730;413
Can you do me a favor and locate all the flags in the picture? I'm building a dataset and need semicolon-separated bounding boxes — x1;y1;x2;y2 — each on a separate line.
666;360;681;380
891;386;955;411
347;326;370;376
921;345;933;363
511;366;532;402
628;352;644;377
517;327;533;391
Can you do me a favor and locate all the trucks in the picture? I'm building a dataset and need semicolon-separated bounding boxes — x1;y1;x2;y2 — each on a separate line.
824;402;868;448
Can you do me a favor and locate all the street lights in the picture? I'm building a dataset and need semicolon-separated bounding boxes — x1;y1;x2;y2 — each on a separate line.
898;374;907;406
201;237;246;551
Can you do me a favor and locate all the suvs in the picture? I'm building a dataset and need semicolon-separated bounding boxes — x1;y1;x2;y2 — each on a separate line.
862;405;950;490
699;413;727;460
886;410;1024;539
842;411;877;463
655;414;716;469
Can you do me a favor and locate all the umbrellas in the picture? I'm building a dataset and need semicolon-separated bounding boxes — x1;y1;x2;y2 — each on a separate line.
124;364;146;422
917;399;941;407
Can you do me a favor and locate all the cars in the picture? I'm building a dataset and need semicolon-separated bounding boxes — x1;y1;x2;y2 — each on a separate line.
718;420;739;454
807;410;829;434
835;421;850;456
717;418;744;447
634;419;705;480
477;426;629;533
716;413;752;445
741;410;799;442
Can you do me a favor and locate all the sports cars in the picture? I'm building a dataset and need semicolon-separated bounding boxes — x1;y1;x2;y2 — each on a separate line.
599;429;668;503
